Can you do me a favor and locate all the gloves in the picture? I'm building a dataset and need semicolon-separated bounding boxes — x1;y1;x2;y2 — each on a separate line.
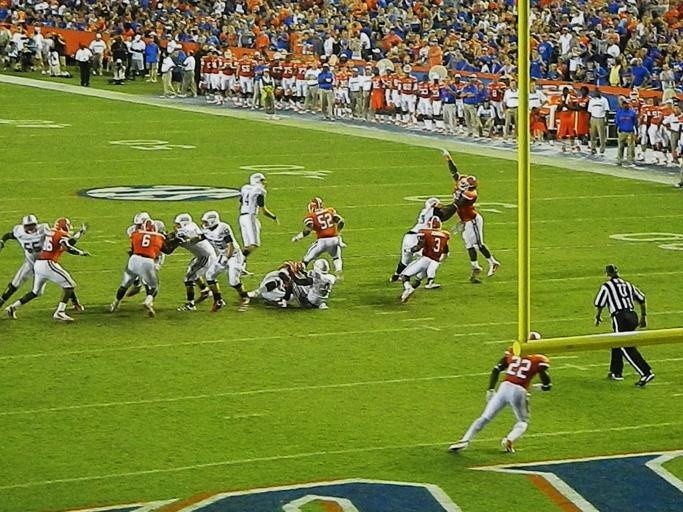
439;148;450;158
486;389;496;403
404;248;412;253
221;256;229;266
595;315;603;326
530;384;542;390
453;196;466;208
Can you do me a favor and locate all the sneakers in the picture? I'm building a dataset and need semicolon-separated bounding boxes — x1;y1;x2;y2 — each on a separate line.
389;270;441;288
111;300;119;312
142;303;155;318
127;286;144;296
635;372;655;386
53;311;74;321
6;305;17;320
75;306;84;311
488;262;500;277
400;288;415;304
177;288;226;311
501;438;515;453
247;291;258;298
470;267;482;281
238;297;251;311
608;372;624;381
271;297;288;308
448;442;468;451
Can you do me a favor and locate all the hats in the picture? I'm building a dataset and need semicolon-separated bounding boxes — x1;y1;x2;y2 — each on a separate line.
530;331;541;339
606;264;619;273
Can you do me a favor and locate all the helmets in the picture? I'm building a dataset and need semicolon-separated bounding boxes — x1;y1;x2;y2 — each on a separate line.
425;198;439;208
134;211;220;233
314;259;330;274
22;215;38;233
308;197;323;214
458;175;477;191
427;215;443;231
54;217;70;233
250;173;266;189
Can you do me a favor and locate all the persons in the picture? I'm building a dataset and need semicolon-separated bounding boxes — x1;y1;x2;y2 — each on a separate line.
399;214;451;302
108;210;246;316
593;263;656;385
1;1;682;168
238;171;281;263
448;331;552;453
440;144;501;277
244;258;306;309
282;258;337;311
389;196;462;284
7;216;96;322
1;212;84;311
290;196;348;282
